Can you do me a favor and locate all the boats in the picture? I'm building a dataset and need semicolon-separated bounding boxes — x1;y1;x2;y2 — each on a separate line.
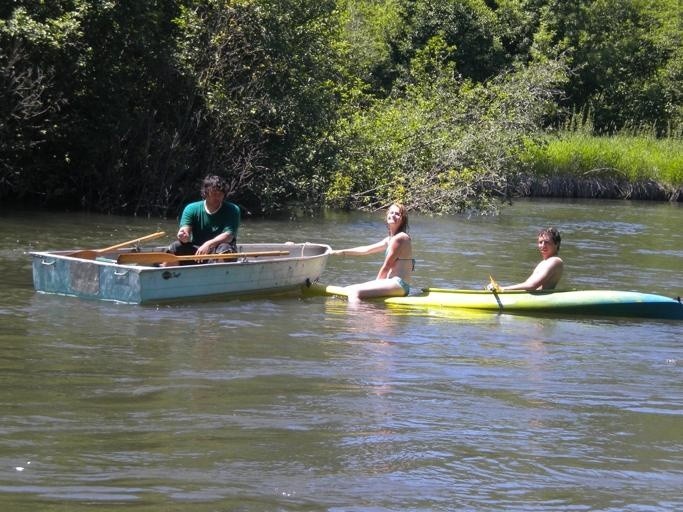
21;243;331;305
306;281;683;322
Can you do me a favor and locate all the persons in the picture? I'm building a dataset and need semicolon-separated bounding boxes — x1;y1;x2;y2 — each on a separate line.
487;225;565;294
159;175;241;267
329;203;414;307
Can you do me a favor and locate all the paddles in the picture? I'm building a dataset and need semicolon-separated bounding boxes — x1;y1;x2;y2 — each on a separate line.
66;232;166;260
117;251;292;268
